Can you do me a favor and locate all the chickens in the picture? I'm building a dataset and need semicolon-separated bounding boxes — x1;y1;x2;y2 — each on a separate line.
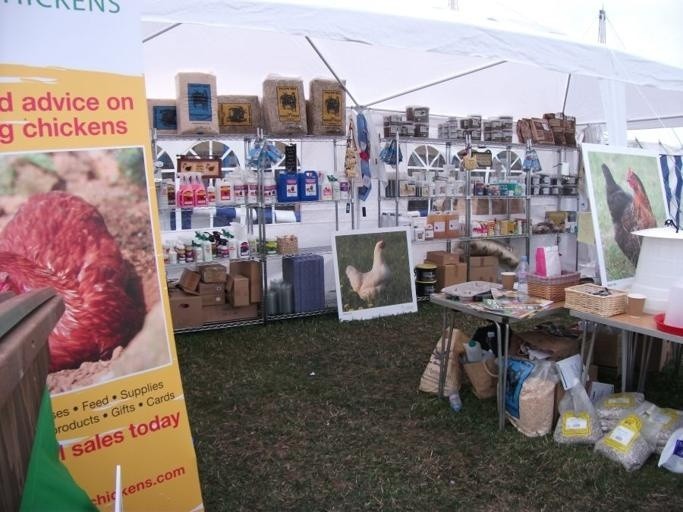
0;189;147;374
601;163;658;270
345;240;392;306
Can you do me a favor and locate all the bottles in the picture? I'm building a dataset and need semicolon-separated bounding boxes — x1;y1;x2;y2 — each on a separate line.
448;386;462;412
517;256;530;302
466;340;481;363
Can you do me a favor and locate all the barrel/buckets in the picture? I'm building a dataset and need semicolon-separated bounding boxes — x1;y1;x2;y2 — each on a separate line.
215;178;233;204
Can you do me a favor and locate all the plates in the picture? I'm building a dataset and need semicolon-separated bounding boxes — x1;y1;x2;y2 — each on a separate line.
441;280;503;301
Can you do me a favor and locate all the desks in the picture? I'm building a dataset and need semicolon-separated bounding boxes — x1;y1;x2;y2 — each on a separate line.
568;306;683;394
429;282;567;430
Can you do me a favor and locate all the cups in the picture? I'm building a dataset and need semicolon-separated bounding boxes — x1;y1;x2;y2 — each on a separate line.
533;178;539;185
627;293;647;319
542;188;550;194
533;187;539;195
552;188;559;194
406;184;417;197
501;272;516;290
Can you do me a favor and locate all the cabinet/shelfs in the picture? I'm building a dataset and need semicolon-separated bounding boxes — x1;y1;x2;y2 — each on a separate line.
376;130;581;301
261;126;355;321
150;126;264;334
